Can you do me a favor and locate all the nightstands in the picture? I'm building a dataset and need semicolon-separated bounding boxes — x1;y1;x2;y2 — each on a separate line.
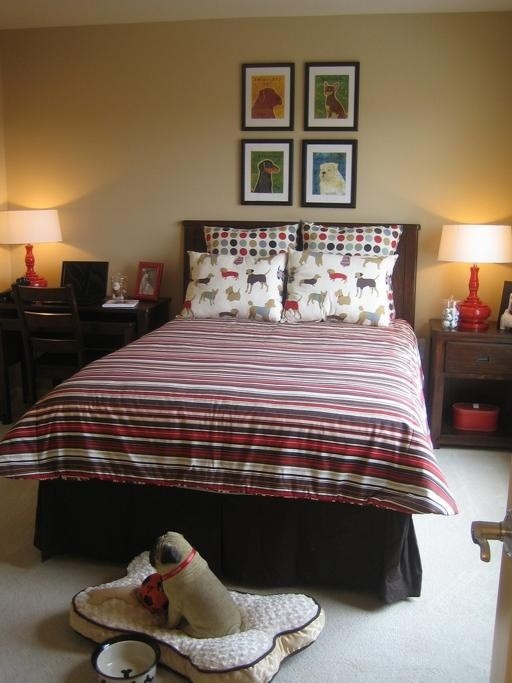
428;318;512;453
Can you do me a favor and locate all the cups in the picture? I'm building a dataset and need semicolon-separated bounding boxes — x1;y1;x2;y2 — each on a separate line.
112;273;129;305
439;295;466;334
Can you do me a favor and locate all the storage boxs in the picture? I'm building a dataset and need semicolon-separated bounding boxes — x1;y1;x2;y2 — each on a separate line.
452;402;500;432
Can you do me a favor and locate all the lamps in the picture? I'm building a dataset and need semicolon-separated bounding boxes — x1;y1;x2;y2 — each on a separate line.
437;223;512;333
0;209;63;288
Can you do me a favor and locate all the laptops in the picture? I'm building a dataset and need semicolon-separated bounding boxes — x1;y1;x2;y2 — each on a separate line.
60;260;109;305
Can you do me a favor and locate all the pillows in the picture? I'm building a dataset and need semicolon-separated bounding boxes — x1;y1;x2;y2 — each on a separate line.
202;223;300;257
279;246;400;330
175;250;287;323
300;220;405;256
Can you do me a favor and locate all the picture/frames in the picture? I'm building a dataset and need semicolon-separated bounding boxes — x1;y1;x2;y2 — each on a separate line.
304;61;360;132
241;62;296;131
301;139;358;208
130;261;164;301
240;139;294;206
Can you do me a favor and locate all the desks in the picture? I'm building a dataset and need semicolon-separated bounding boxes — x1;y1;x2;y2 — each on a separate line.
0;295;172;425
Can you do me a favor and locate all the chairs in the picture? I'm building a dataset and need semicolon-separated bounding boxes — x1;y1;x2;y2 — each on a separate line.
10;282;84;404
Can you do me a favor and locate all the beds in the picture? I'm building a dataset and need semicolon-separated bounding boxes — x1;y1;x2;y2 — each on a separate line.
0;220;442;607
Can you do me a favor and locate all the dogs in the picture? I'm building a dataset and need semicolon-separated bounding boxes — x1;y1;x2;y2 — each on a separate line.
354;272;381;299
251;157;282;193
219;309;239;319
360;255;387;270
306;290;328;309
283;295;303;320
322;80;349;120
245;268;273;294
199;288;219;307
277;265;285;282
197;253;219;266
181;293;197;318
195;273;215;287
225;286;240;301
288;266;299;284
254;253;278;266
251;87;283;120
318;160;348;197
220;267;240;281
325;311;347;323
356;305;385;327
299;274;322;288
247;299;277;322
148;531;249;641
335;289;351;306
327;268;347;284
138;268;156;296
